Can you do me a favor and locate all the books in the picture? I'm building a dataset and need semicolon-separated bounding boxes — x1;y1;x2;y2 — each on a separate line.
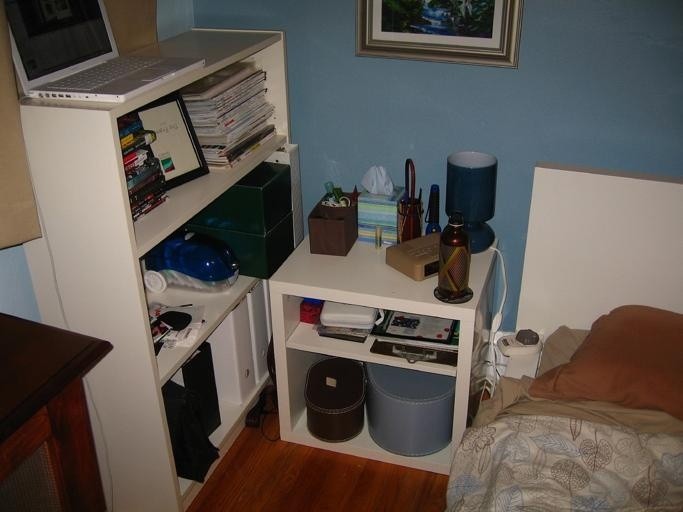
118;60;276;222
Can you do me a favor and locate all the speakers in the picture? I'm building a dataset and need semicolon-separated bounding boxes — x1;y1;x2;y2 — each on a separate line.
182;162;292;234
171;212;294;279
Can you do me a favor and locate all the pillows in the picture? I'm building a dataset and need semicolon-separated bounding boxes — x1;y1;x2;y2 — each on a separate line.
529;305;683;421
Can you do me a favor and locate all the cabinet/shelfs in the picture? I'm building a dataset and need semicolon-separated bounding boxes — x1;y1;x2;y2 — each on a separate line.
20;29;301;512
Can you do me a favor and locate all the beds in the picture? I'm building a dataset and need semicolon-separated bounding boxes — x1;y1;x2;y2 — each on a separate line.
482;163;683;512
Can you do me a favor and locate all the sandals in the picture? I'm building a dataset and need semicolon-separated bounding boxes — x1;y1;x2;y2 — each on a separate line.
245;385;279;442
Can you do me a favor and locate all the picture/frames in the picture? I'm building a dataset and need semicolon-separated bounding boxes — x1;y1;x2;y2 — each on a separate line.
356;0;524;69
132;97;209;193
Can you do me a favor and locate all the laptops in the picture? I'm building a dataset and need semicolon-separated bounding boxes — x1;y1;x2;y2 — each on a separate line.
4;0;205;103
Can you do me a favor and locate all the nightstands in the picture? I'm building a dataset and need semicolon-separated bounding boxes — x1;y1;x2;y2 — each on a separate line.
269;234;500;476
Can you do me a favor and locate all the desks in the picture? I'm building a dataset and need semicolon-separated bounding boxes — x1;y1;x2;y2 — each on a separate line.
0;313;114;512
